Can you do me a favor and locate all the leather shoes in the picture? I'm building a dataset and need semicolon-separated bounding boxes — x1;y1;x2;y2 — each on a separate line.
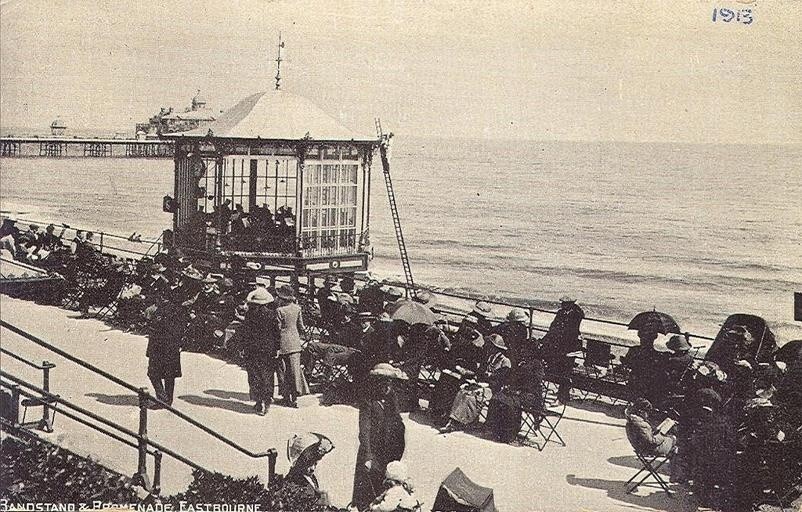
439;421;453;432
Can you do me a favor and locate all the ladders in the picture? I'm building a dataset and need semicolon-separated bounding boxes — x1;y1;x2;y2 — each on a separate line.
372;117;417;298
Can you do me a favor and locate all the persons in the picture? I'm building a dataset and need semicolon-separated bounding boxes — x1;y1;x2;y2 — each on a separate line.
284;431;335;491
350;361;410;512
625;323;800;512
1;219;584;442
197;199;293;235
369;460;425;511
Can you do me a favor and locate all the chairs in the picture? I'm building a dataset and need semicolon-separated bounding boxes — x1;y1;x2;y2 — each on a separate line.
0;209;802;512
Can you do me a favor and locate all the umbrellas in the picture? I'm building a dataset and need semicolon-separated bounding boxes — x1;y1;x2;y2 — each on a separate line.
630;306;680;336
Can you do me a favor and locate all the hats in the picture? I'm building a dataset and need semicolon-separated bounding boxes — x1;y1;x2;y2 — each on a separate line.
247;287;274;304
324;270;577;349
665;335;691;351
368;362;411;380
288;430;334;466
275;284;297;300
